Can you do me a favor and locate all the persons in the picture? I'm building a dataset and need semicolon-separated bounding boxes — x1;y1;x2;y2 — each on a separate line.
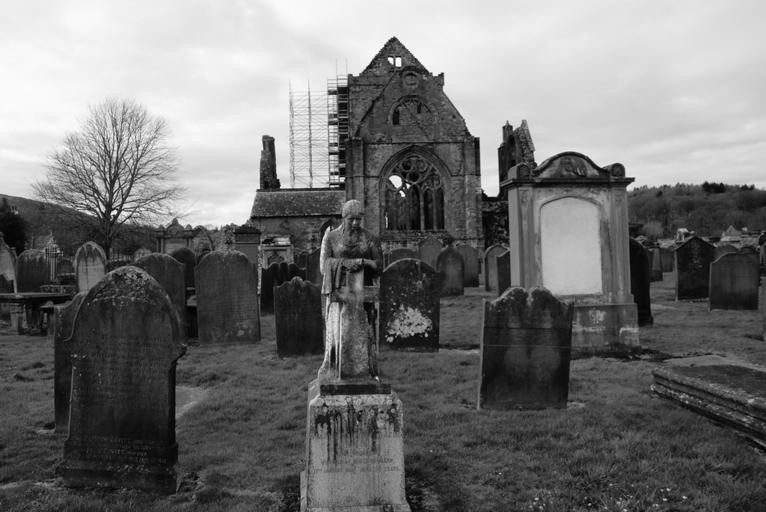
317;199;384;388
